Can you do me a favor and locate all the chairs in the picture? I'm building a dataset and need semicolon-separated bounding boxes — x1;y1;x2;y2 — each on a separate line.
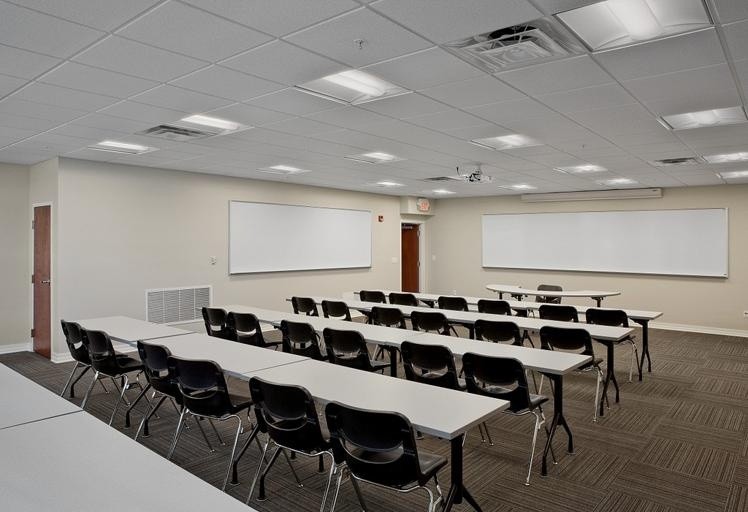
369;306;407;363
585;308;641;383
321;300;353;321
389;293;418;307
323;328;391;374
227;311;283;350
201;307;227;339
167;354;268;493
462;352;558;486
539;325;611;423
438;296;469;312
410;311;451;336
133;339;218;460
360;291;386;303
400;340;486;448
477;299;511;316
281;320;344;360
60;319;160;426
538;304;580;323
528;284;563;334
244;376;335;512
325;400;449;512
474;319;523;346
291;296;320;317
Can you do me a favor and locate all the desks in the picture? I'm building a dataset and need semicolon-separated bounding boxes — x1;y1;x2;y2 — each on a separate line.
485;284;621;307
66;315;198;428
474;313;635;416
511;300;663;381
354;289;440;308
136;332;312;487
433;294;536;317
285;297;398;324
358;302;494;339
243;358;511;512
272;313;424;377
385;333;592;475
196;303;309;351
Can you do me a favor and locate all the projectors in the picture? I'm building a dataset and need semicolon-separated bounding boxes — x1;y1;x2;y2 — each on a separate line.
463;167;493;185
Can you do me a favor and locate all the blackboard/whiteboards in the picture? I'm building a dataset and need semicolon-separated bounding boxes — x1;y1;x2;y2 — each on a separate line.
481;207;729;279
227;199;372;275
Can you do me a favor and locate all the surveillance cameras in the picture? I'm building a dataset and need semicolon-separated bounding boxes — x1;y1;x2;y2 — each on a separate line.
353;37;368;50
